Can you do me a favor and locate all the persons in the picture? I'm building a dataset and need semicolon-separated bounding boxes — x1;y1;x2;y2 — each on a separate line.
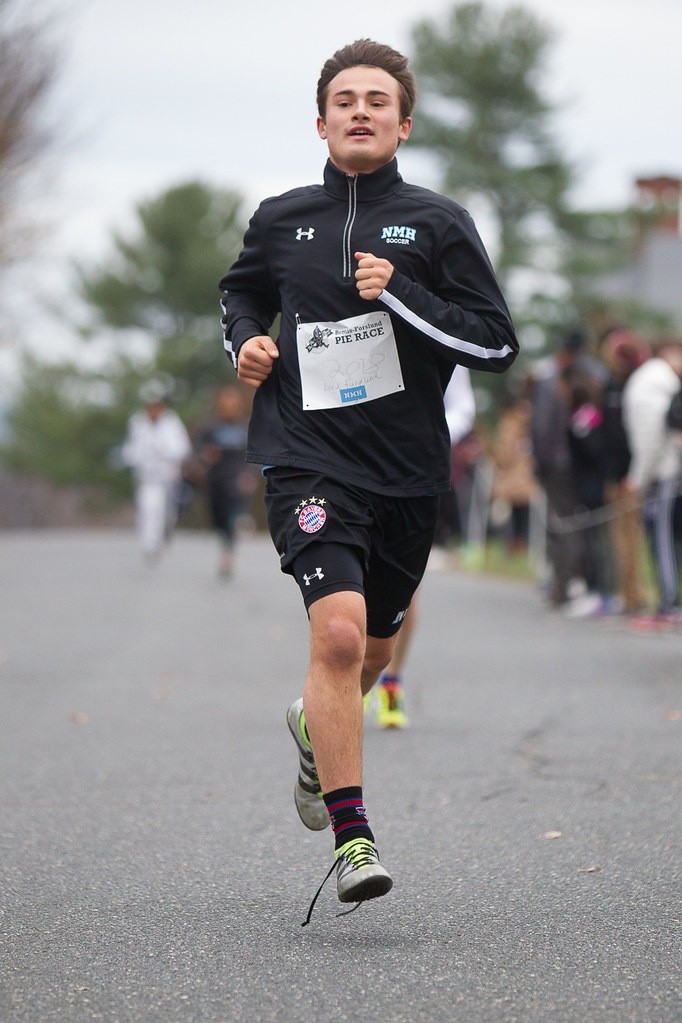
220;39;519;904
125;304;682;637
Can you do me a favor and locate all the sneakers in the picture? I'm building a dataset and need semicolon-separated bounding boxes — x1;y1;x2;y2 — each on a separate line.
332;842;394;906
286;695;335;833
377;673;407;731
